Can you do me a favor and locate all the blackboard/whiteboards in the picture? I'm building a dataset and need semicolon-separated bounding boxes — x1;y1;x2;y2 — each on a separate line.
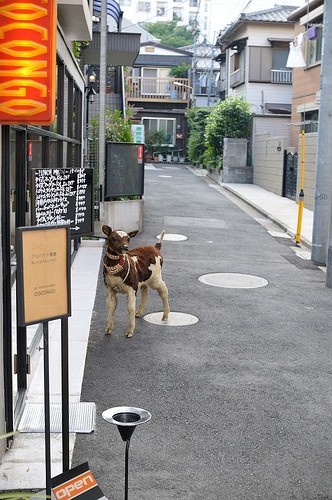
32;167;94;239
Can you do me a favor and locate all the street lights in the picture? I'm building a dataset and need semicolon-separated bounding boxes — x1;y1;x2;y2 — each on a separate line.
172;81;195;109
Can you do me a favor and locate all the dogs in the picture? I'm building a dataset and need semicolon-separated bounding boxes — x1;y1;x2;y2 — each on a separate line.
100;223;170;338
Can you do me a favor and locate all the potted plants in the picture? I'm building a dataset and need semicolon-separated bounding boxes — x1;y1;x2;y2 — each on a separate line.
90;99;251;238
